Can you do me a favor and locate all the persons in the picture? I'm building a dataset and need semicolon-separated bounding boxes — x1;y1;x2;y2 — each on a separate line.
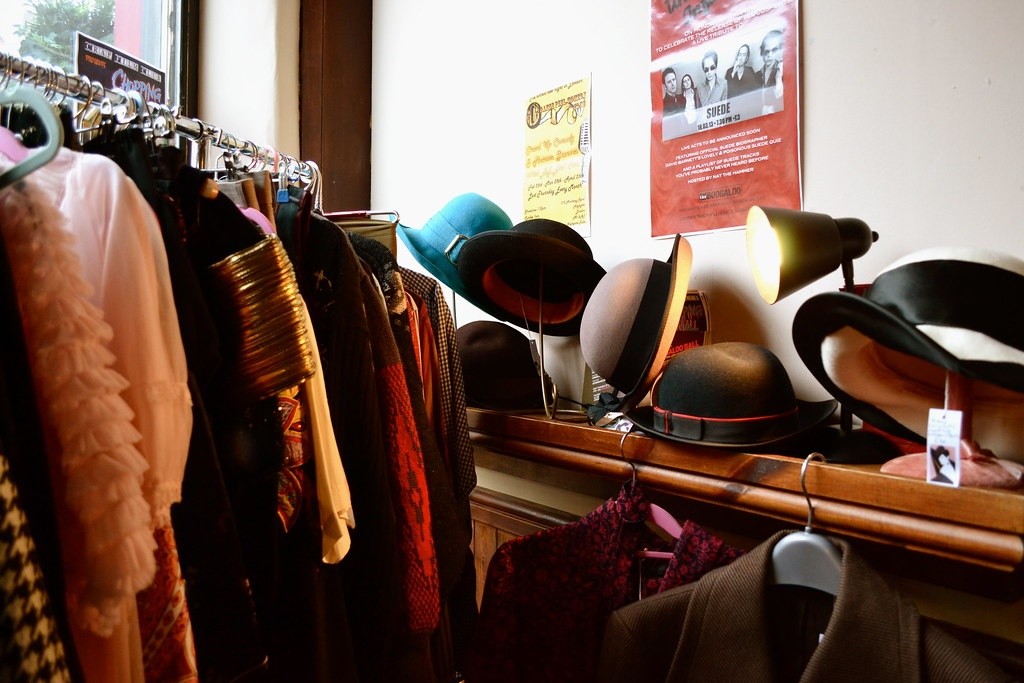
679;73;702;111
755;29;786;91
661;66;685;116
695;50;728;109
726;43;761;99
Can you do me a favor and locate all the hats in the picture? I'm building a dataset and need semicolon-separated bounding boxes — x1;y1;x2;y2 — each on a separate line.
397;191;1024;465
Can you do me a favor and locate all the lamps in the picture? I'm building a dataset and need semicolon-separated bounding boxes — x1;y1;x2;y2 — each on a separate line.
744;201;907;465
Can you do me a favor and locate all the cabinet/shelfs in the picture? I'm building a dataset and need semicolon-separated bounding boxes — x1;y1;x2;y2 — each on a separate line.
467;406;1024;677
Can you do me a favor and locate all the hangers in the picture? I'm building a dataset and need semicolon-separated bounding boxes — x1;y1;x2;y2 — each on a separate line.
1;53;442;319
